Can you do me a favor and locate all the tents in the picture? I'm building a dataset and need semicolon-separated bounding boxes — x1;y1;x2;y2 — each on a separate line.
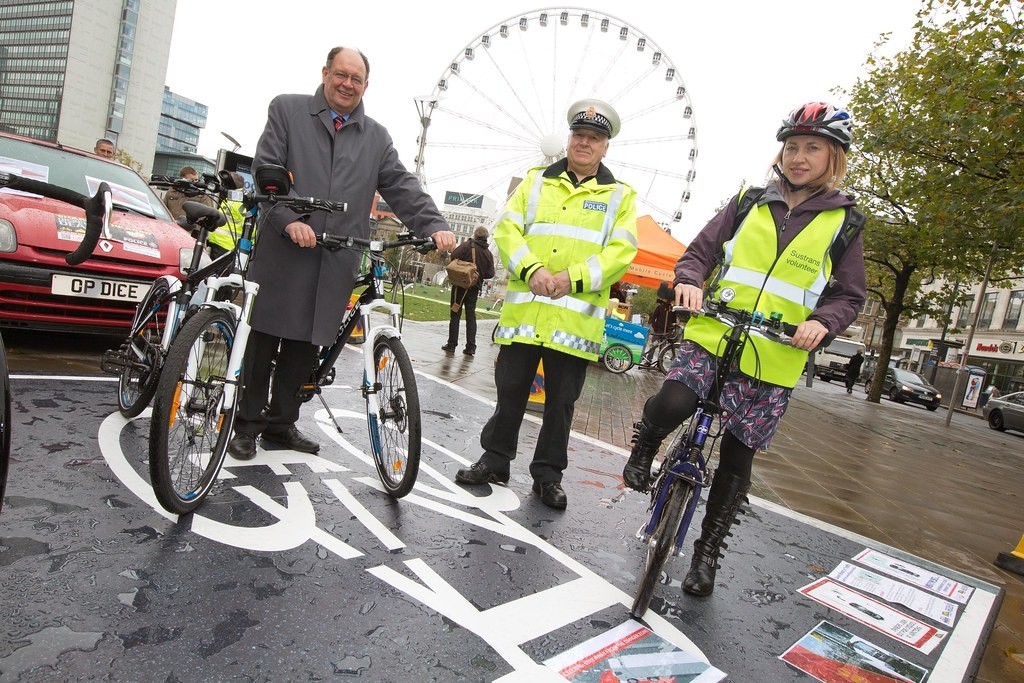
619;216;707;291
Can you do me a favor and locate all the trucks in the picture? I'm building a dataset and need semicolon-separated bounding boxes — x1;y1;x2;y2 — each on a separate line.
805;324;868;385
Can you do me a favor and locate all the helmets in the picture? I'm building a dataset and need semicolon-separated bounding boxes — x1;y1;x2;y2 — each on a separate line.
776;101;852;153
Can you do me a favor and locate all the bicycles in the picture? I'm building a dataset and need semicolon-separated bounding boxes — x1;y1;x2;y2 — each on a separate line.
299;221;444;499
99;158;353;520
629;278;837;625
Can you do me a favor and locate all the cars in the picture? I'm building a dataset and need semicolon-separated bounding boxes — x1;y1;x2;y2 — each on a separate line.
981;391;1024;435
863;367;941;410
0;126;219;353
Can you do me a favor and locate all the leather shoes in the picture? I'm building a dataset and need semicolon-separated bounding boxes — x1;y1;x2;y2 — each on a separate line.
455;461;510;485
262;426;320;453
532;479;567;509
229;431;257;459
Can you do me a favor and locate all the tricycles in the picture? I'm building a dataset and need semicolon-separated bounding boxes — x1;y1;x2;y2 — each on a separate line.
603;293;685;377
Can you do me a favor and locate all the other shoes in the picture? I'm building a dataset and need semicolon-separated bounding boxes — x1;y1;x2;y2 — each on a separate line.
464;349;475;354
441;343;455;351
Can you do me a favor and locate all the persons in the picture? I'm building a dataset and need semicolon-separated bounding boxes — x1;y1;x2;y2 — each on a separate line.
209;172;258;278
358;268;369;276
417;269;432;286
889;563;920;577
624;102;866;596
164;166;211;223
988;384;1001;400
852;641;922;679
94;139;113;158
643;298;677;372
455;99;637;511
849;603;883;620
966;378;980;400
442;226;495;354
845;348;864;394
226;46;455;461
610;281;624;302
392;270;397;283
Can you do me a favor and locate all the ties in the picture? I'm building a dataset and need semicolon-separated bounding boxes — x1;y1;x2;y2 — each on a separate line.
334;114;343;130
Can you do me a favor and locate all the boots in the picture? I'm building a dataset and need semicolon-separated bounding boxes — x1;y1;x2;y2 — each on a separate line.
681;467;752;596
623;394;673;490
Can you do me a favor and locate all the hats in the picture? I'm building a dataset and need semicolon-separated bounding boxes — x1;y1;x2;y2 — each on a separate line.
475;225;489;238
566;99;621;140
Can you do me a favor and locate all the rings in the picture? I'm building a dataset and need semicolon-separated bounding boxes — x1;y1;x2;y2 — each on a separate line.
810;332;817;337
555;290;560;295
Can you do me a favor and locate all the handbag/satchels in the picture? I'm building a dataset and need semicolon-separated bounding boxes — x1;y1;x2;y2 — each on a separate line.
446;258;476;288
